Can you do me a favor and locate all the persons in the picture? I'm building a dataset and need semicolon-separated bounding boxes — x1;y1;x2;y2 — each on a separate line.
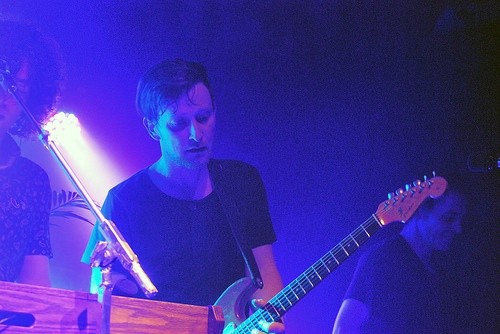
332;176;468;334
81;58;286;334
0;19;64;286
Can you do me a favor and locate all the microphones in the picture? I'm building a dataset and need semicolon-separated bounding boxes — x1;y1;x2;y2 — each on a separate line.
0;57;17;95
466;151;500;173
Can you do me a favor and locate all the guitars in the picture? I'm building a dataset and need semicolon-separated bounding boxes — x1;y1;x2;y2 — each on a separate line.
206;171;447;334
